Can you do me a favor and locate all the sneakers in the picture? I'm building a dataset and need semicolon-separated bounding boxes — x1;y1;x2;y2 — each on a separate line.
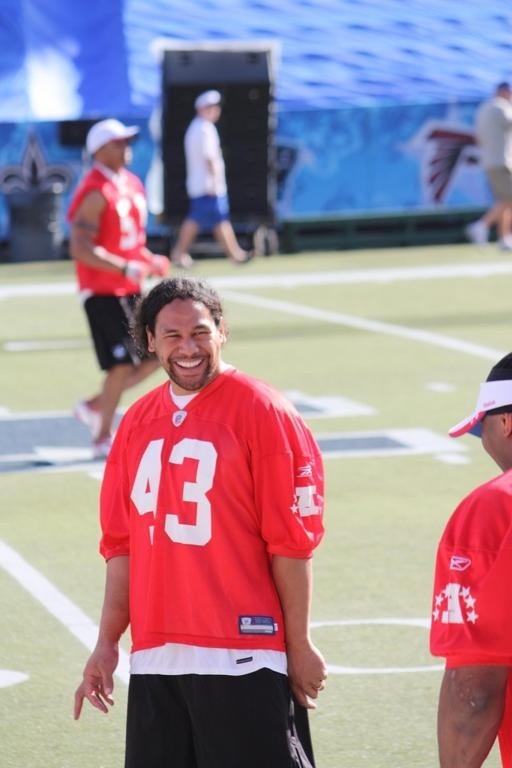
74;396;114;463
464;222;512;252
170;248;257;271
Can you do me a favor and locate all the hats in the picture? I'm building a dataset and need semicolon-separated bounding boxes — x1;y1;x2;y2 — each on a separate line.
446;345;512;440
190;84;222;111
84;116;141;153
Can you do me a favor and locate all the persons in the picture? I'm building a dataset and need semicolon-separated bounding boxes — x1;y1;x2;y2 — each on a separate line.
64;115;173;460
75;278;328;767
170;89;258;268
462;83;512;253
427;353;512;768
